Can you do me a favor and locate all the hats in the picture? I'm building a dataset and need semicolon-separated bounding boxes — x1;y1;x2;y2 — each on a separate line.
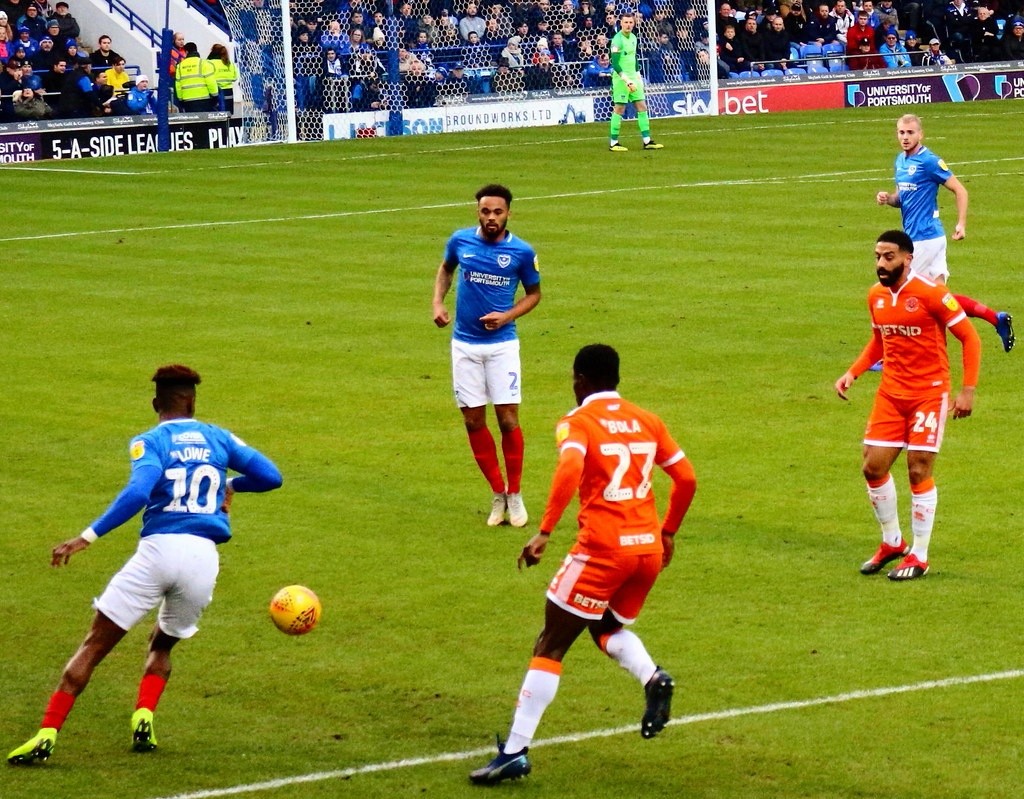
905;31;917;40
507;36;521;50
373;26;386;42
453;61;463;70
1010;15;1024;30
886;28;898;38
929;38;939;44
497;57;509;68
436;63;450;77
746;9;758;15
859;37;870;46
791;3;801;11
765;8;775;15
537;38;547;48
0;4;148;99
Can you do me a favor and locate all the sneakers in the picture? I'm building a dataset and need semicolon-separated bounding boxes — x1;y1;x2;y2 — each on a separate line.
886;554;929;580
640;666;675;739
506;486;530;528
868;361;884;371
486;486;508;526
7;727;58;765
128;708;158;752
470;743;532;789
860;538;911;574
996;311;1016;353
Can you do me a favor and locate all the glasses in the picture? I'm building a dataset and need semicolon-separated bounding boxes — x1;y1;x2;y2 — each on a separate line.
581;2;589;6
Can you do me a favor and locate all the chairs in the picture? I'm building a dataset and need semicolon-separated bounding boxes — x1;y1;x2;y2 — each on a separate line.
196;0;849;108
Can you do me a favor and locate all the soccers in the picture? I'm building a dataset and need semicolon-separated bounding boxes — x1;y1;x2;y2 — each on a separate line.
270;584;321;636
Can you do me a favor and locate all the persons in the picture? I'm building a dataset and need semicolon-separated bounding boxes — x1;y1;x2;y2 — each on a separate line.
430;182;546;529
868;114;1016;372
469;341;701;787
834;228;981;580
0;0;1023;123
608;10;664;153
6;364;285;767
